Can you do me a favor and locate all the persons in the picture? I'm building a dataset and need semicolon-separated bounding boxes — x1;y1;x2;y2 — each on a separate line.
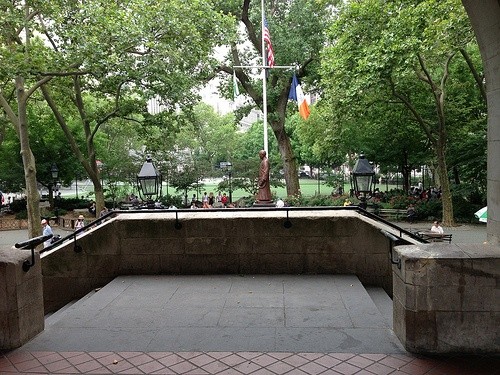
276;197;290;207
408;187;442;201
130;193;139;206
368;188;388;203
257;149;271;200
431;221;443;242
407;203;416;224
155;200;162;209
41;219;54;247
418;181;423;189
0;191;3;211
75;215;85;235
344;199;351;206
100;207;108;217
339;187;342;195
7;196;11;204
190;192;227;209
169;204;177;210
89;202;97;216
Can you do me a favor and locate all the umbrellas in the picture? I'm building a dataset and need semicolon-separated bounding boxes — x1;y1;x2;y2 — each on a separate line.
474;206;487;223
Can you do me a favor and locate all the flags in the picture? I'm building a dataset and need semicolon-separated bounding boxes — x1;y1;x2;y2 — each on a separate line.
263;15;275;68
290;73;310;121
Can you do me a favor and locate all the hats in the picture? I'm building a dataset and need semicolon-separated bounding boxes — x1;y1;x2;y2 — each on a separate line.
78;215;84;219
41;220;47;224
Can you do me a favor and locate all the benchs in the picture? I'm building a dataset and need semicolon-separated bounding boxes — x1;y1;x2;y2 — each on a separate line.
404;228;452;244
369;209;418;224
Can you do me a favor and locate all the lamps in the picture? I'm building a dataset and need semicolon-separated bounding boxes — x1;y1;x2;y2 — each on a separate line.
137;156;162;208
350;150;376;209
